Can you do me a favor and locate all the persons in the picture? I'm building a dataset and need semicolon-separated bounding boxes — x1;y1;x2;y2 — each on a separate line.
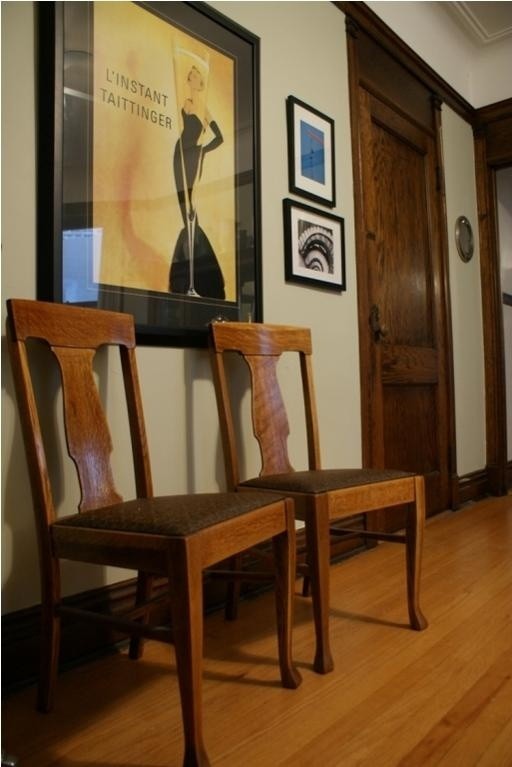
173;59;224;297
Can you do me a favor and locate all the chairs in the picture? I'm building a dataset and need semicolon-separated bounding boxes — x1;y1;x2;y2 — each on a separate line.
207;317;431;678
2;292;307;767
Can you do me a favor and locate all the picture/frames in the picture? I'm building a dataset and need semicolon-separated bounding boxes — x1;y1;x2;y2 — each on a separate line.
31;2;272;348
284;90;338;207
281;198;349;294
